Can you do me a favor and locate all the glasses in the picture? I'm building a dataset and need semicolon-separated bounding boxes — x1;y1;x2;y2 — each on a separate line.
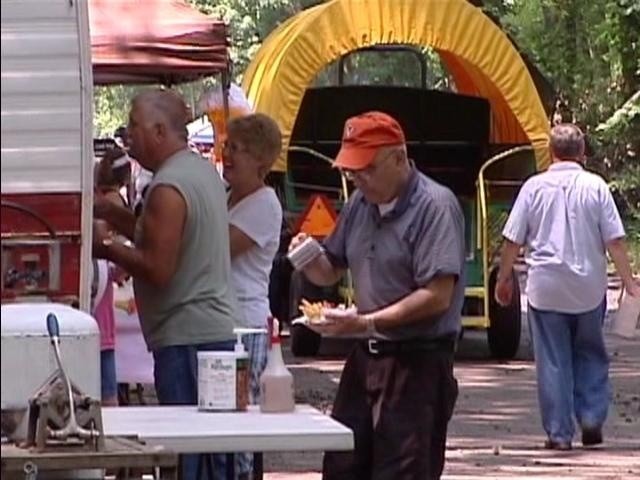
344;148;398;180
219;141;247;153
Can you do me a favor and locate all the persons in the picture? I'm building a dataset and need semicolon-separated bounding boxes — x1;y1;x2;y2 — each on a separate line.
89;125;161;405
494;121;640;452
215;113;286;480
281;110;468;480
91;88;238;480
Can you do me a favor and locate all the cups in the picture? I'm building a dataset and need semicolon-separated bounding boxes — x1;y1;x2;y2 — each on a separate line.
285;236;325;271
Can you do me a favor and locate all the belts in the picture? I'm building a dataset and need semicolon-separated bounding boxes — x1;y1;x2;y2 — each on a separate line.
367;337;447;356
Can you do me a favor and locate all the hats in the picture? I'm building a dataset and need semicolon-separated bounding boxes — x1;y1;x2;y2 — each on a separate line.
331;111;406;170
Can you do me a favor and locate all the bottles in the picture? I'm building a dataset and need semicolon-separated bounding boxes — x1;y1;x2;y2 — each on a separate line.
612;293;640;337
230;326;266;411
259;336;293;413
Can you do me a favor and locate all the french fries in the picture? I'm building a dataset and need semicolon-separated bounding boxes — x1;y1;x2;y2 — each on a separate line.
299;298;328;319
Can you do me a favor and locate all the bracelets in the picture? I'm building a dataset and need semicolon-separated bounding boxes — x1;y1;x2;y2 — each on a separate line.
496;272;514;287
366;312;376;339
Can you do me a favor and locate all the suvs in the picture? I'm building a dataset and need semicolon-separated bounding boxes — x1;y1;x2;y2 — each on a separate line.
240;2;557;357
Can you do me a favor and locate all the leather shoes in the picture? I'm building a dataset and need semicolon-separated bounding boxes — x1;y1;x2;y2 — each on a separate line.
545;441;572;451
585;427;607;447
